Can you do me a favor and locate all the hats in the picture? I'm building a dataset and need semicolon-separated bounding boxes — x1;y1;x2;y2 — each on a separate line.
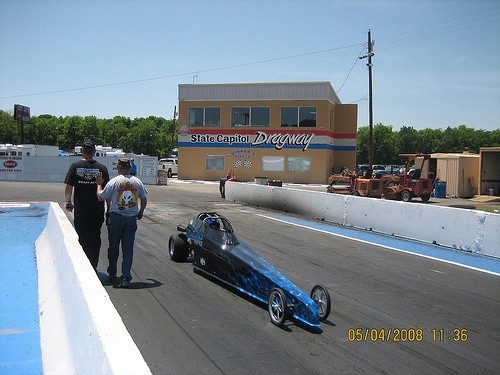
118;158;131;166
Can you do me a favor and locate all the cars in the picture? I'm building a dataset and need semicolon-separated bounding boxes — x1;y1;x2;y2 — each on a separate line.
372;164;385;170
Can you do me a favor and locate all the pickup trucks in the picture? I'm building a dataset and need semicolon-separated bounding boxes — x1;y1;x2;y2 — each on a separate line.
158;158;179;177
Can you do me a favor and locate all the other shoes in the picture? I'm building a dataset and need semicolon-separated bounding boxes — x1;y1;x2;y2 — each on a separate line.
107;267;116;277
122;280;129;287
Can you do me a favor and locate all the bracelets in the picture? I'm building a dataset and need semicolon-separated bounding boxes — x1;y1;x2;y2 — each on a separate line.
65;200;71;204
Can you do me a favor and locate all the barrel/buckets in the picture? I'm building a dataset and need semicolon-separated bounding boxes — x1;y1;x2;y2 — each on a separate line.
220;178;240;198
255;177;268;185
269;181;282;187
488;187;495;195
435;180;446;198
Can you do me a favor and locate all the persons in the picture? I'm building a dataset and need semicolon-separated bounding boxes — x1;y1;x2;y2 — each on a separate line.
64;142;110;273
95;158;148;286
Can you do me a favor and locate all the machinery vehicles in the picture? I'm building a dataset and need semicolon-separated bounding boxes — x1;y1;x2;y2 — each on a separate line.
327;153;432;203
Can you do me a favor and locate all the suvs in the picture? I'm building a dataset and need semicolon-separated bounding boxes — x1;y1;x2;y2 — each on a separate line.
359;165;369;174
386;165;399;170
